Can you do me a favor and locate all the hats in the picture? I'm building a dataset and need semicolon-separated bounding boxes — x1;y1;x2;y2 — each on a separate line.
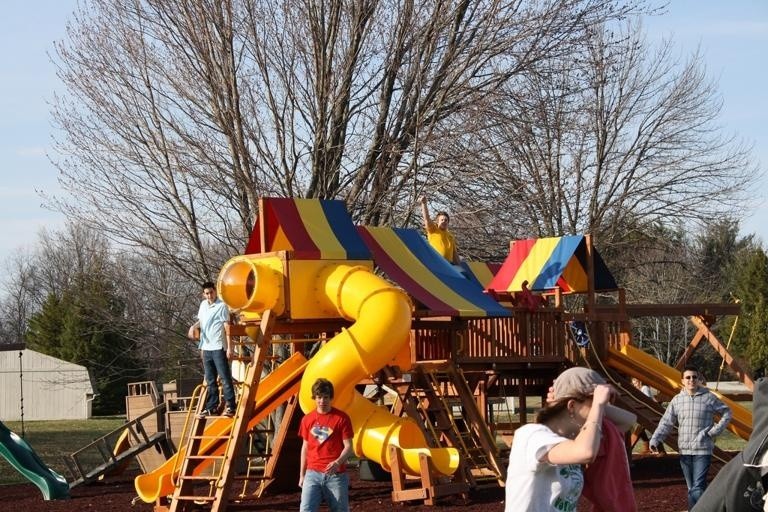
545;366;606;409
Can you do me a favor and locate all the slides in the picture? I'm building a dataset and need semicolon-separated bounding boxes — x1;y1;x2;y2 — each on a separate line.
298;264;460;479
606;332;757;439
135;350;313;503
0;421;70;500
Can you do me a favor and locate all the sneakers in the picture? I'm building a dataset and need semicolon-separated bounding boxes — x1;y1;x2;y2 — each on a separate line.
222;408;235;417
199;409;217;417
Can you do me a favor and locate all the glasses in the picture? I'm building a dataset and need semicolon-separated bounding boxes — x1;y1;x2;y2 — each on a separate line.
683;375;698;380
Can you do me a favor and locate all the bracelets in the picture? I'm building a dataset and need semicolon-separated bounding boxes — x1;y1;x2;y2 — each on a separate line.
334;459;340;466
578;419;603;432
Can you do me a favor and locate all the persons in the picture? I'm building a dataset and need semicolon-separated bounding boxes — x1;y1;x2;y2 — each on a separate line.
296;377;354;512
420;195;460;265
648;366;734;512
502;366;637;512
186;280;236;418
